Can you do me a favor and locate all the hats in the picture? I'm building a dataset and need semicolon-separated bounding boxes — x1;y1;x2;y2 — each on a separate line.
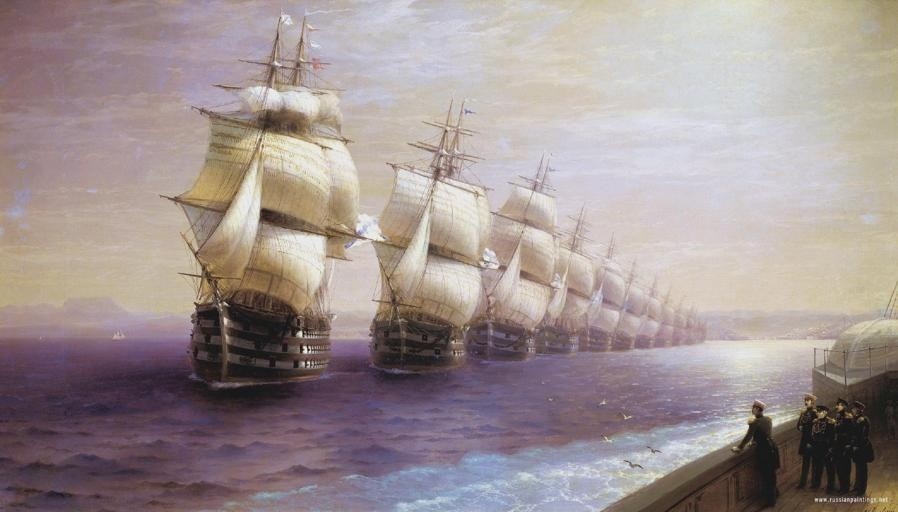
836;397;848;406
752;398;769;411
854;399;866;410
815;404;829;412
805;392;818;401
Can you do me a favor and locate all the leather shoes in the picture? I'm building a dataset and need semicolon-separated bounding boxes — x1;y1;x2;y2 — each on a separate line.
794;484;864;498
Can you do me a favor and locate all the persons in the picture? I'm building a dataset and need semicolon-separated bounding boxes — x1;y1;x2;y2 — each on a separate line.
732;399;781;506
791;393;820;489
807;406;835;492
847;400;872;496
831;397;851;495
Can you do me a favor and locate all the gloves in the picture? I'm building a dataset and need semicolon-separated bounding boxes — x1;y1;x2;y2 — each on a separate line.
732;446;741;451
